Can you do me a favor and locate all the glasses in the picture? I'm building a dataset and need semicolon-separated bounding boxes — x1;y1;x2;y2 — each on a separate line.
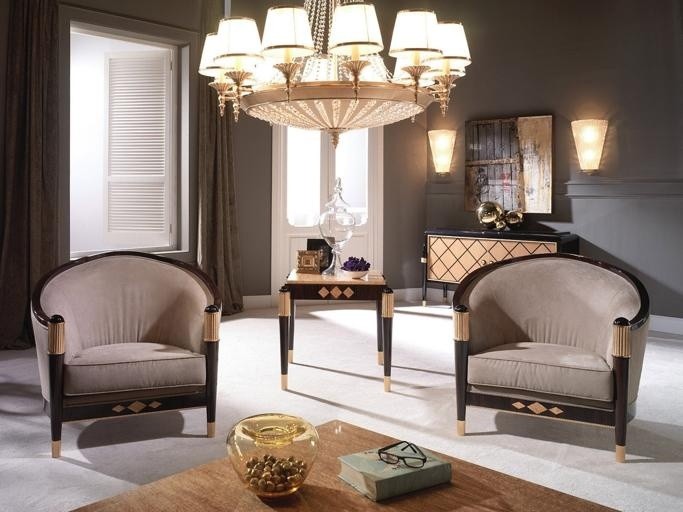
375;436;428;471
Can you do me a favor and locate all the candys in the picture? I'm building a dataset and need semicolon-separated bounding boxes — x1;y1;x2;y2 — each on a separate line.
244;453;307;491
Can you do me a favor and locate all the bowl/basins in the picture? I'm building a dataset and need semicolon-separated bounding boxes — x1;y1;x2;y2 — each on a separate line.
342;269;369;279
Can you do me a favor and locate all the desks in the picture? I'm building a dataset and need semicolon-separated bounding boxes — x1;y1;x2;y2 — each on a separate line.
420;226;569;306
69;419;619;512
278;267;392;394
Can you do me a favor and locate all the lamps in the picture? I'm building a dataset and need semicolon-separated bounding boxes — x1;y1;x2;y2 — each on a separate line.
427;129;456;179
569;118;608;181
197;1;473;147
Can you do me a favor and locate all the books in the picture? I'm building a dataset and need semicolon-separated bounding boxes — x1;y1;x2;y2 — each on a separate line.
337;443;452;502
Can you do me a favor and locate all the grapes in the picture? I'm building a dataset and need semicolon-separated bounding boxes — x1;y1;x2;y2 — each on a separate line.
341;256;370;271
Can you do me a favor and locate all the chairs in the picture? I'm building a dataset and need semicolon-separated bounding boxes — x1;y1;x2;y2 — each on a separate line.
31;249;225;457
451;253;648;466
